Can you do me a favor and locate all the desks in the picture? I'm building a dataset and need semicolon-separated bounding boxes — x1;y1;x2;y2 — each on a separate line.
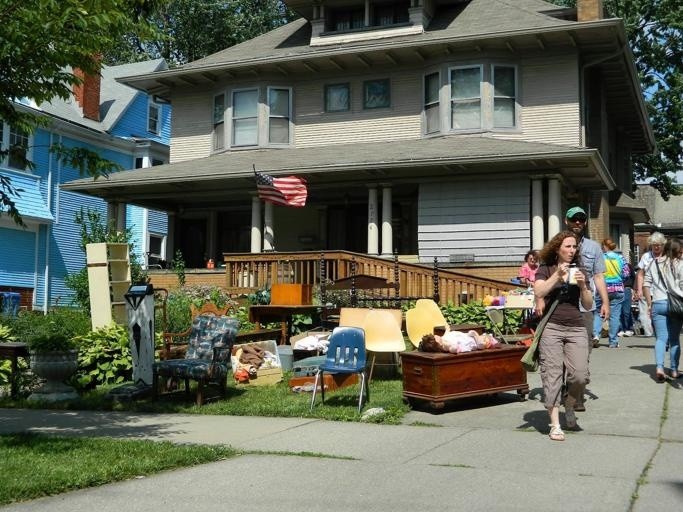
248;305;321;346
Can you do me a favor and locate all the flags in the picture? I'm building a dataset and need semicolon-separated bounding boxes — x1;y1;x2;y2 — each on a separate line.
252;164;308;209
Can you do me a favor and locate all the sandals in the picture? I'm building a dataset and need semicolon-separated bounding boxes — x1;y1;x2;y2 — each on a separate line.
550;424;565;441
565;398;576;428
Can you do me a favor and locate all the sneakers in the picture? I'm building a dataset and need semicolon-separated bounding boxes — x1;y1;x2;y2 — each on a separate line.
574;402;585;411
592;337;600;346
623;330;634;337
617;331;624;337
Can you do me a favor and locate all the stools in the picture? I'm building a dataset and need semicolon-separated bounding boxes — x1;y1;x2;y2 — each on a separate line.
0;342;30;400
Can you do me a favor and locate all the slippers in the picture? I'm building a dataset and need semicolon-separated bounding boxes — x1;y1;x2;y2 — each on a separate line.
656;374;668;383
668;373;683;381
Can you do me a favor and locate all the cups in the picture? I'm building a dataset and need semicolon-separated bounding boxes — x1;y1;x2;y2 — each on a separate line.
499;297;504;305
567;264;579;285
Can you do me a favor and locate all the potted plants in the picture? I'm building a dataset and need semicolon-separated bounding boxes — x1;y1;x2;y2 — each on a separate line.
27;328;78;401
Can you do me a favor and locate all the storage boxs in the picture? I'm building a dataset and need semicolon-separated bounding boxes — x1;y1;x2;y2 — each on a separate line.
434;325;487;338
247;367;282;387
269;284;311;305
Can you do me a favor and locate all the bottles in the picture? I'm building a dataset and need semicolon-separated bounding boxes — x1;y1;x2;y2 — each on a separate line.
207;258;214;268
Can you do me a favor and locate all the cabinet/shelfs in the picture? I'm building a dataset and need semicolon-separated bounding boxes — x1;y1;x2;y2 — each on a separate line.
399;344;534;414
86;242;131;331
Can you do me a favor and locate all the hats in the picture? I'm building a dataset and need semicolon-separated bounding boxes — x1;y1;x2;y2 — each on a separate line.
566;206;586;219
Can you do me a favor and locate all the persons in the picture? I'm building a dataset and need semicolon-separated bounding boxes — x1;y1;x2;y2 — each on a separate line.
519;204;682;442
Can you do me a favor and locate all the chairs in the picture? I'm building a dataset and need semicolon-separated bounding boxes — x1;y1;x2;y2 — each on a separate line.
369;307;404;379
150;315;240;408
158;302;233;361
339;307;367;350
405;298;448;349
485;308;533;348
308;325;370;412
153;288;169;335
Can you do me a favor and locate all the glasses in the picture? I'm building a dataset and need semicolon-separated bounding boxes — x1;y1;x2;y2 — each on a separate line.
569;216;587;223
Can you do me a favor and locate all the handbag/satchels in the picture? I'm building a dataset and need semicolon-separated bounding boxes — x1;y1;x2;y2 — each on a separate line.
666;291;683;323
520;326;542;372
233;366;249;382
